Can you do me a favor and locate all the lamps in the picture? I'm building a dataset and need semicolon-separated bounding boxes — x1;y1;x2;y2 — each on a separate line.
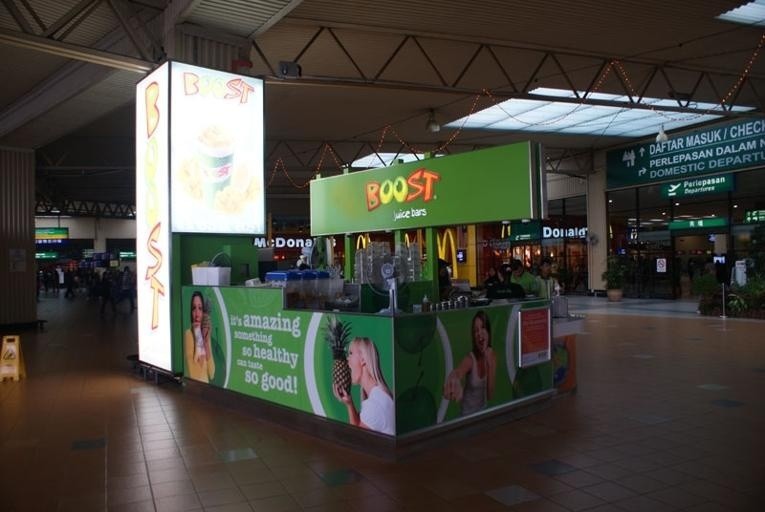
655;128;667;143
426;108;441;135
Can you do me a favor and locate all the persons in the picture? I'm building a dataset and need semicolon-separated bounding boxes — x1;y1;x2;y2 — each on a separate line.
676;252;731;287
329;335;396;438
35;261;134;318
438;257;460;300
182;290;217;383
299;255;311;271
508;258;536;297
530;251;587;295
441;309;498;417
482;267;499;288
532;258;555;301
484;264;525;299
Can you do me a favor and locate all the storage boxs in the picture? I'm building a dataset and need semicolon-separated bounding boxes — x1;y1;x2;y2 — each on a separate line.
190;267;231;288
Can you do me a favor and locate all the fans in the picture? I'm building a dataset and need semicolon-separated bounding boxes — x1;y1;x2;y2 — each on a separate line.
363;237;417;315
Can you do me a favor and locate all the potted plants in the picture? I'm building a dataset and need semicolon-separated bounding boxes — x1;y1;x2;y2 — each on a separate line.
601;260;629;302
691;272;721;304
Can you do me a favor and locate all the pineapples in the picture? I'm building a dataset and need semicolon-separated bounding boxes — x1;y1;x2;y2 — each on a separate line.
321;315;352;397
201;299;212;332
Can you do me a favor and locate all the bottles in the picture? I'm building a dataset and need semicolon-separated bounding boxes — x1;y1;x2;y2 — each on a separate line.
422;294;430;312
430;295;468;311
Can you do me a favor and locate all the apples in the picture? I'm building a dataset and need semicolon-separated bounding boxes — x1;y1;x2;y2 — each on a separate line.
553;368;576;394
398;315;436;352
513;367;542;398
396;386;437;432
537;363;552;389
551;346;568;367
